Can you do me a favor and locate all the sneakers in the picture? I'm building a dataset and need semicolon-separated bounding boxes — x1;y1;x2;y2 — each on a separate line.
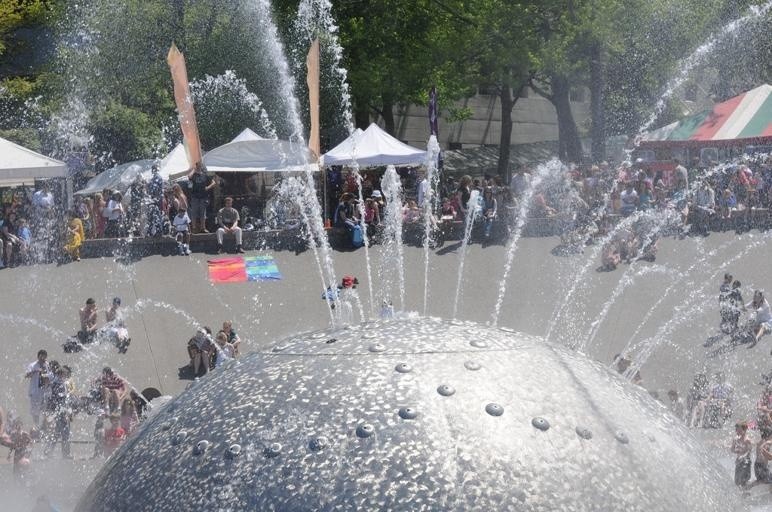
216;247;223;254
237;247;244;253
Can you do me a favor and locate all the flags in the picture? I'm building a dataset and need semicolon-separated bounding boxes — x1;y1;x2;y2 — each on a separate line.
208;253;282;282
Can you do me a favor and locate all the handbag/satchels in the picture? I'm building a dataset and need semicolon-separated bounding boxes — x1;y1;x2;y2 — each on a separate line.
353;225;363;248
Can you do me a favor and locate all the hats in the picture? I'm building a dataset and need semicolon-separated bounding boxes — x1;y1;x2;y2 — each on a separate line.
371;189;382;197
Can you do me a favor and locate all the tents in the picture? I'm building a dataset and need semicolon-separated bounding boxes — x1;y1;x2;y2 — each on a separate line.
319;123;431;228
0;136;74;211
72;129;322;195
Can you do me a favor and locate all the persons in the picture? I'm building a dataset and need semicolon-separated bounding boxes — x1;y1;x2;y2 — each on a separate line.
0;152;772;273
0;298;147;479
614;273;772;488
188;320;242;381
